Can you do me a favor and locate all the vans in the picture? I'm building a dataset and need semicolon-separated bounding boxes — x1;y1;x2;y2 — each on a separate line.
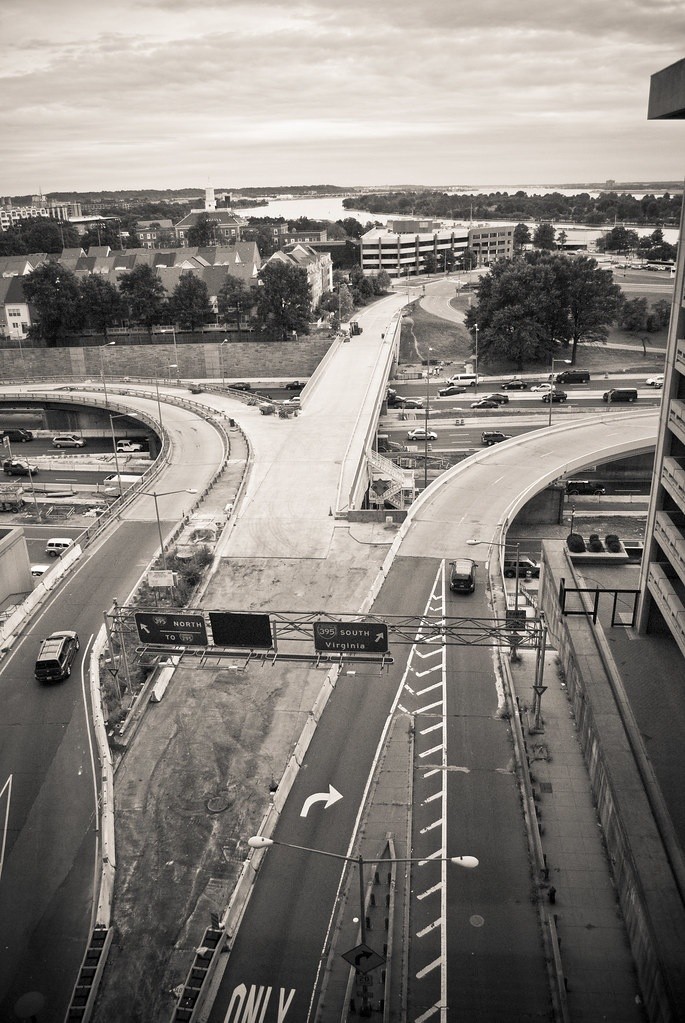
445;374;479;386
647;375;664;388
3;428;34;443
604;388;638;402
557;370;591;384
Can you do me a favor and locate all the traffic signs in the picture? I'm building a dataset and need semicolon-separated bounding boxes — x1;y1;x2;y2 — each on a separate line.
311;622;388;655
134;612;209;648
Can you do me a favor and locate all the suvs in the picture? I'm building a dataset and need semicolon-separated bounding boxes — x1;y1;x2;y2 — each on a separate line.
449;558;479;594
504;554;543;578
45;538;74;557
53;434;87;448
566;480;607;496
117;439;144;453
3;458;38;477
30;565;49;581
479;431;512;446
34;630;79;684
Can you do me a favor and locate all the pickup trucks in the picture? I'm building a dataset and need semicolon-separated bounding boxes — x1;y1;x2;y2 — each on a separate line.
286;381;306;391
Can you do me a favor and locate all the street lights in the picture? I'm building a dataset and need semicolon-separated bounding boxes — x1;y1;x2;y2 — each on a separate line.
105;485;198;571
474;323;480;394
161;328;182;387
220;339;228;388
465;539;524;662
109;411;138;504
100;342;116;406
246;834;482;1015
551;358;573;426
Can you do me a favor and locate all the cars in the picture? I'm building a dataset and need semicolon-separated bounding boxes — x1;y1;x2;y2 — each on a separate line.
228;382;250;391
387;379;572;409
407;429;438;440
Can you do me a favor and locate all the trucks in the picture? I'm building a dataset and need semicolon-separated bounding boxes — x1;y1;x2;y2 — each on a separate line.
0;485;26;514
103;474;147;497
